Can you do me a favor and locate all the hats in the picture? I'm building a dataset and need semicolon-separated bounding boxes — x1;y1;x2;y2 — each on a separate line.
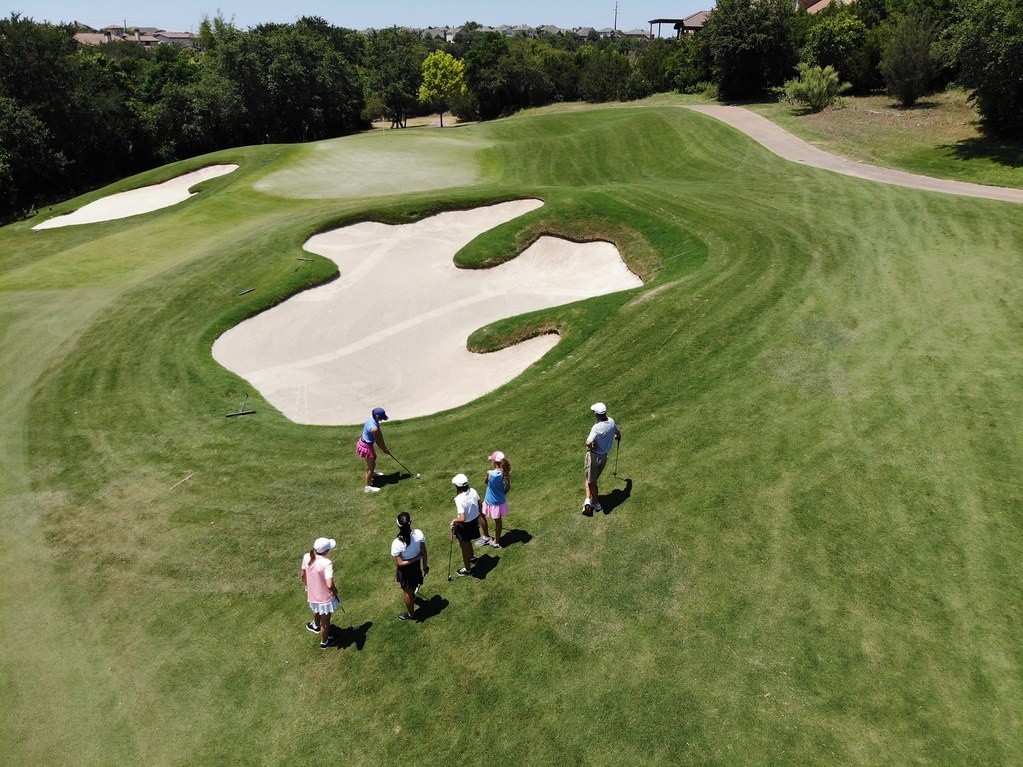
314;537;336;553
372;408;388;420
452;474;468;487
591;403;606;414
488;452;505;462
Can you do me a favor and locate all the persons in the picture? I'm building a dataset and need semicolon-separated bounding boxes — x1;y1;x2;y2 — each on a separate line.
391;511;429;620
451;473;482;576
301;537;340;650
582;402;622;515
356;408;390;493
475;451;511;548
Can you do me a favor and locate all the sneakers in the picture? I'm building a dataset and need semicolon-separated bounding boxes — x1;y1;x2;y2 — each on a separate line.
457;568;473;576
306;621;321;634
470;558;478;564
398;612;418;622
489;540;499;548
475;536;490;546
320;636;336;648
582;499;590;514
595;504;602;510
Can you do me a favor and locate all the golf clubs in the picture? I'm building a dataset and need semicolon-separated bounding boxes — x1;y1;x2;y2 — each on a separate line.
336;597;356;635
614;439;620;476
413;573;427;599
447;524;454;582
388;453;414;478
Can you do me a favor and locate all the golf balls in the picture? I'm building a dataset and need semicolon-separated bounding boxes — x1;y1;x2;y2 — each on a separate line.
417;474;420;478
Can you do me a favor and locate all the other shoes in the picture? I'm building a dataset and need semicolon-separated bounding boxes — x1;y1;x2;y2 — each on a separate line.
364;486;380;492
371;472;383;476
